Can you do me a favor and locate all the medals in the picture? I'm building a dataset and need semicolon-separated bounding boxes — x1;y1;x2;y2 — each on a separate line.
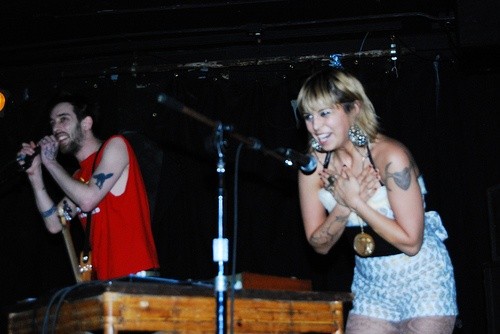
352;233;376;257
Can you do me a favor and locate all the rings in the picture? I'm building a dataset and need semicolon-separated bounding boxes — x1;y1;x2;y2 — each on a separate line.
327;175;336;186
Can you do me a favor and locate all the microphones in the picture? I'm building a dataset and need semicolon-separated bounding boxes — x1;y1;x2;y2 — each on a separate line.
17;146;48;170
248;138;316;173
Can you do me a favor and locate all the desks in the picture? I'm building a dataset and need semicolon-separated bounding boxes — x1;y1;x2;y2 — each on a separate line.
2;280;356;333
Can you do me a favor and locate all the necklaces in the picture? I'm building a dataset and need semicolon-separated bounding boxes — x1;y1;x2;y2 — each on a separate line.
360;147;369;172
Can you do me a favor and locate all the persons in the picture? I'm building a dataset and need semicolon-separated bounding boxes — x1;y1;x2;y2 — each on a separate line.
296;55;460;334
18;96;161;296
